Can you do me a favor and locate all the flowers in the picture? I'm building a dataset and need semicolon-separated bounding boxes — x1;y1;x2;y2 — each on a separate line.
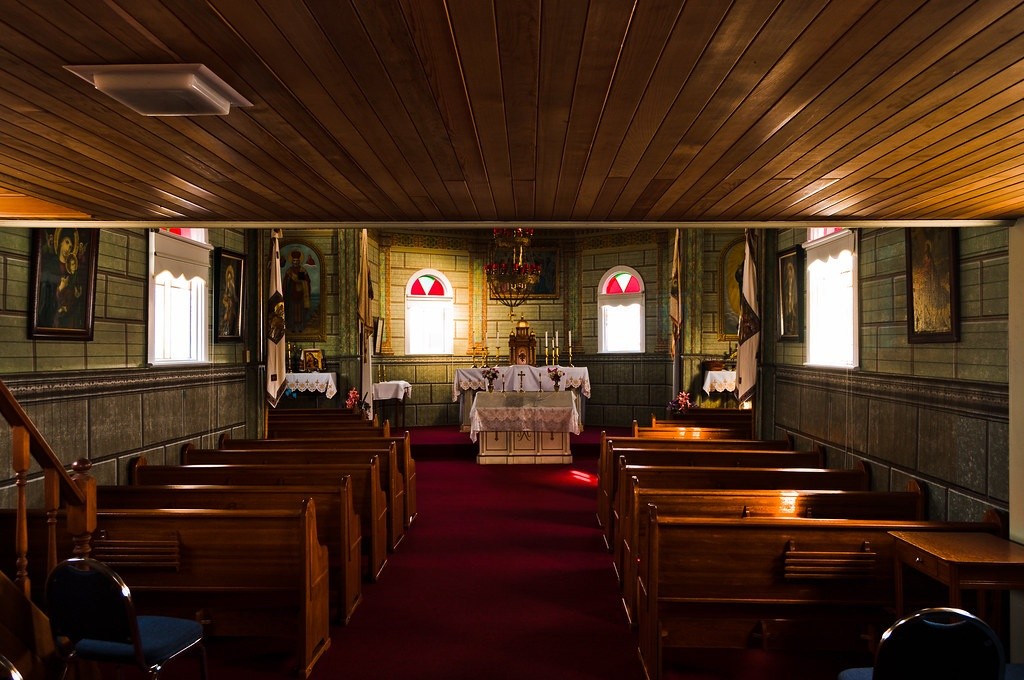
665;392;692;416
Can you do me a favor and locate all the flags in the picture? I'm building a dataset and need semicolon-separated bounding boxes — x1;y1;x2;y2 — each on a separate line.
670;228;683;359
356;229;374;364
267;238;288;409
734;228;760;410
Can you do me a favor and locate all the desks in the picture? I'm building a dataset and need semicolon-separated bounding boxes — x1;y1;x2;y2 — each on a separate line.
702;369;736;395
452;368;591;432
888;530;1024;627
286;372;338;408
372;379;411;428
469;389;579;464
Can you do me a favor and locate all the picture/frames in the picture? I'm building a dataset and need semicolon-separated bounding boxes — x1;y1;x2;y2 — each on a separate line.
265;238;328;344
28;227;100;340
213;246;247;344
302;348;324;371
371;317;386;355
716;235;747;342
775;245;806;343
485;244;560;300
905;227;960;346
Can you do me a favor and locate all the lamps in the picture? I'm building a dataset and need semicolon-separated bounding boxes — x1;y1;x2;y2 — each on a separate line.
63;63;254;119
482;224;541;307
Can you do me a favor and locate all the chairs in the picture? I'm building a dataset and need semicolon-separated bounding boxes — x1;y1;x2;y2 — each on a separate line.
43;557;209;680
838;606;1010;680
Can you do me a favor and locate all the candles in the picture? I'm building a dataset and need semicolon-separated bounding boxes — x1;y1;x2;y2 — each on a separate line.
544;330;559;350
568;330;573;348
496;331;500;347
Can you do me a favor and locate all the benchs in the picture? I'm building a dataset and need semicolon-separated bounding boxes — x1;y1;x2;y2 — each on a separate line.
1;426;417;677
595;412;1004;680
